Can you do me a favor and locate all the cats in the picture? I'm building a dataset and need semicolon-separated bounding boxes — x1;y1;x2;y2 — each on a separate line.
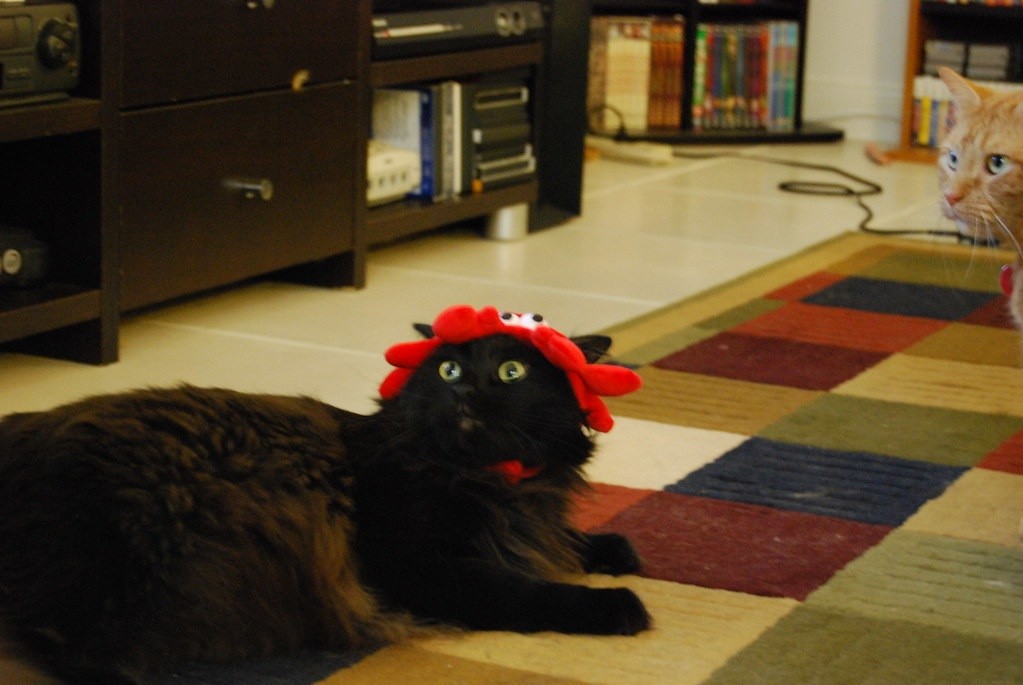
936;64;1023;327
0;304;653;685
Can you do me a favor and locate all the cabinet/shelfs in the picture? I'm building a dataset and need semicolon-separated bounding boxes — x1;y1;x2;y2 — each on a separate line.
589;0;844;145
1;0;591;362
879;0;1023;163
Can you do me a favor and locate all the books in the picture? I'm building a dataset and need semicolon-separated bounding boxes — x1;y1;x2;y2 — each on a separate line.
370;80;463;202
587;15;800;136
924;39;1010;82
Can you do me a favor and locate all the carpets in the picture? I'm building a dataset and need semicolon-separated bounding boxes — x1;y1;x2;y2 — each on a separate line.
0;225;1023;685
